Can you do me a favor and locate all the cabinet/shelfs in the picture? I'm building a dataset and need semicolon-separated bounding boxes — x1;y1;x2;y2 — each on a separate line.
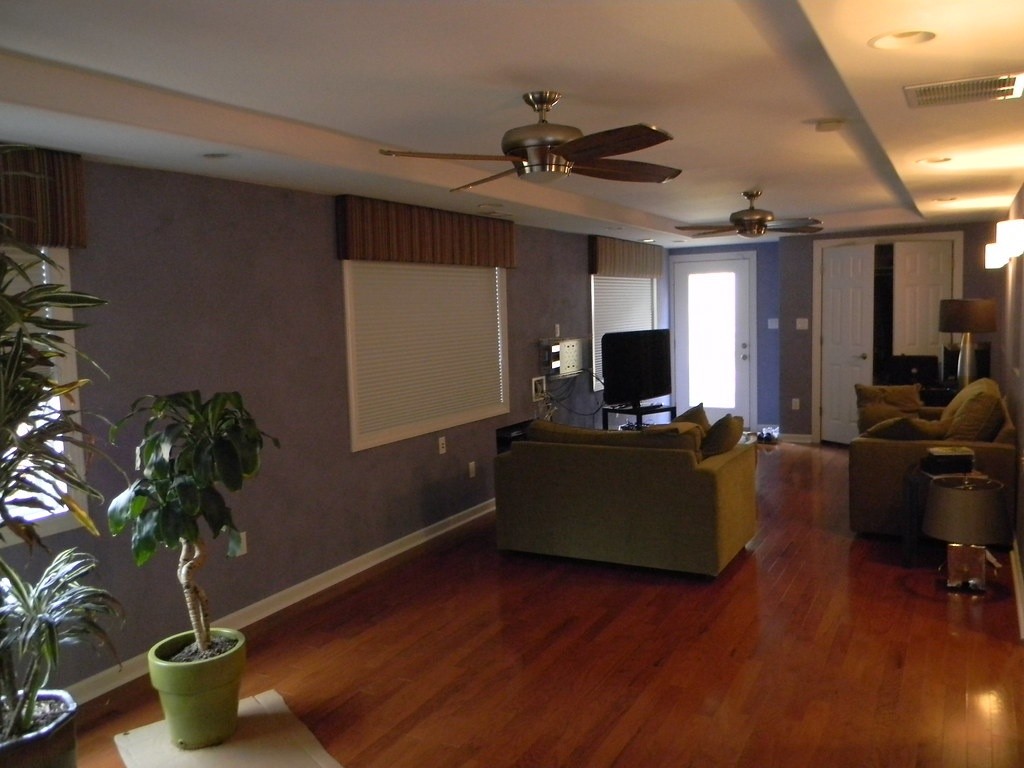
817;240;954;446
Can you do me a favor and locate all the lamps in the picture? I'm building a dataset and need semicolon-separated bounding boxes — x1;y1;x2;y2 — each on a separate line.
939;299;1000;389
922;473;1013;587
985;217;1024;272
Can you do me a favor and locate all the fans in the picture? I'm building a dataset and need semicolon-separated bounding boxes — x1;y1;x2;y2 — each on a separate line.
379;89;683;194
675;189;824;238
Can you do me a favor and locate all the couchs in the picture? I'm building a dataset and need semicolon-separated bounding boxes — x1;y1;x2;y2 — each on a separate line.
493;420;756;577
849;383;1016;533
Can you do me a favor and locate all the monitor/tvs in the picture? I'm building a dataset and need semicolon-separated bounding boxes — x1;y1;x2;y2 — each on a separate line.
602;329;673;411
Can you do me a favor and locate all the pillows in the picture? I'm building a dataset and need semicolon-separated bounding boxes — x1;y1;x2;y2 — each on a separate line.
673;402;743;458
855;377;1007;441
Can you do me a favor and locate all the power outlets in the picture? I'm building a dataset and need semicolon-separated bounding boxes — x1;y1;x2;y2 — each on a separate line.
439;437;446;454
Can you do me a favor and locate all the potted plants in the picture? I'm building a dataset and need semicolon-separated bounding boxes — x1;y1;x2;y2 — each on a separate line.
0;214;131;768
107;389;282;750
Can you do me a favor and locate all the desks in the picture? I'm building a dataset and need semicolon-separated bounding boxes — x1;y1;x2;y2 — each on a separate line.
602;403;676;430
